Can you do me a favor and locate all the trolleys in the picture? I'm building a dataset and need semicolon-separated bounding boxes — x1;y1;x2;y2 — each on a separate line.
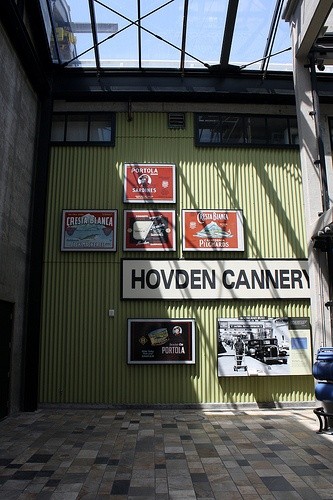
234;349;248;371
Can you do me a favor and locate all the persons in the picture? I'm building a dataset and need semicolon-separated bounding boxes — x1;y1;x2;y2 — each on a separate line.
139;175;150;186
235;335;244;369
171;328;183;344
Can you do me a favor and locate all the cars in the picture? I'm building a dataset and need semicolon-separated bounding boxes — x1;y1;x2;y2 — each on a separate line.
247;339;289;364
